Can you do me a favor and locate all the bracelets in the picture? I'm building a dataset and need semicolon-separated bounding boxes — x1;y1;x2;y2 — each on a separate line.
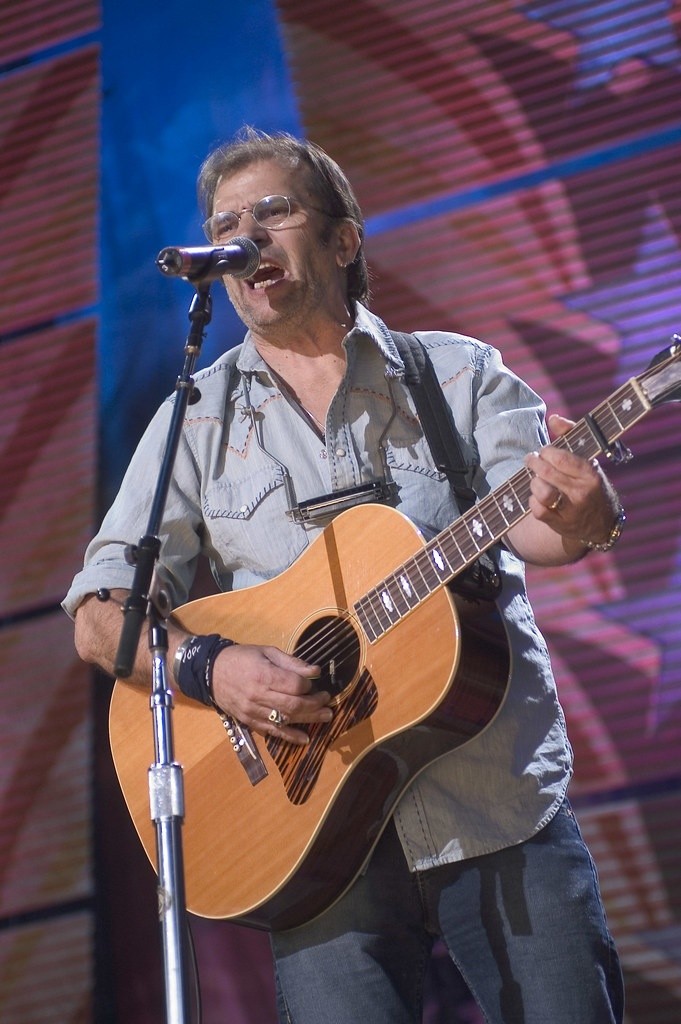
172;633;236;706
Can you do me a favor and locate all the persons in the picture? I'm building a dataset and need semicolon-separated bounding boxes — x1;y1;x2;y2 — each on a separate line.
73;135;627;1022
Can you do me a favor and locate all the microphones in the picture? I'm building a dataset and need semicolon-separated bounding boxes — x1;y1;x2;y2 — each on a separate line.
154;236;261;279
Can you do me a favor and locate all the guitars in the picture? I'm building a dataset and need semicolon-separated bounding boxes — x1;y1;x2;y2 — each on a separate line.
106;333;681;937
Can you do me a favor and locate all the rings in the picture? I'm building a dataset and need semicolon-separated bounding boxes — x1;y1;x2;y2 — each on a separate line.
268;709;290;728
547;491;567;511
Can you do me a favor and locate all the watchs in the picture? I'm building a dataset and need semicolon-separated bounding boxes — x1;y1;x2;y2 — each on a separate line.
578;505;626;551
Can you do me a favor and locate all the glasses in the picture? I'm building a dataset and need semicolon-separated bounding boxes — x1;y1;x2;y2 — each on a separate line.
201;195;335;246
